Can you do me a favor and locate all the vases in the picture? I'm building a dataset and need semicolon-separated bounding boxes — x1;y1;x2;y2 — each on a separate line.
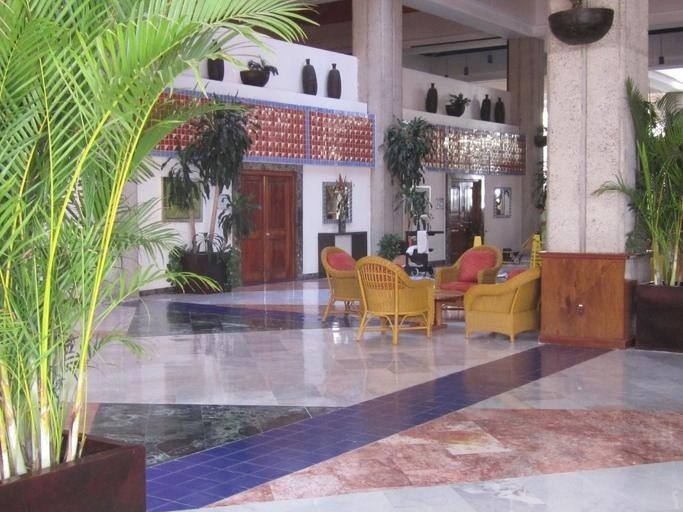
338;215;345;233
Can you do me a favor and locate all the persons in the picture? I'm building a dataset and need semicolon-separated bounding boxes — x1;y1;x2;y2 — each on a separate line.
535;204;545;248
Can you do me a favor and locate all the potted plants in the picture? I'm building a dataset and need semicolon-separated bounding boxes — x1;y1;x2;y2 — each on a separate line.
207;37;226;83
534;126;547;148
589;76;683;355
162;89;252;293
0;0;321;512
444;93;471;117
239;59;279;87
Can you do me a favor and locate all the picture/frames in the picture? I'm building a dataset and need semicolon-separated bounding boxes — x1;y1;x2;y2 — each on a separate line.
321;182;352;225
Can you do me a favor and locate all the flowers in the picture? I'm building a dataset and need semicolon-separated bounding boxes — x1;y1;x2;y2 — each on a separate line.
335;173;349;215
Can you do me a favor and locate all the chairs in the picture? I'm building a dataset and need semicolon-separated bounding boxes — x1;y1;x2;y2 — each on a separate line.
321;244;540;345
